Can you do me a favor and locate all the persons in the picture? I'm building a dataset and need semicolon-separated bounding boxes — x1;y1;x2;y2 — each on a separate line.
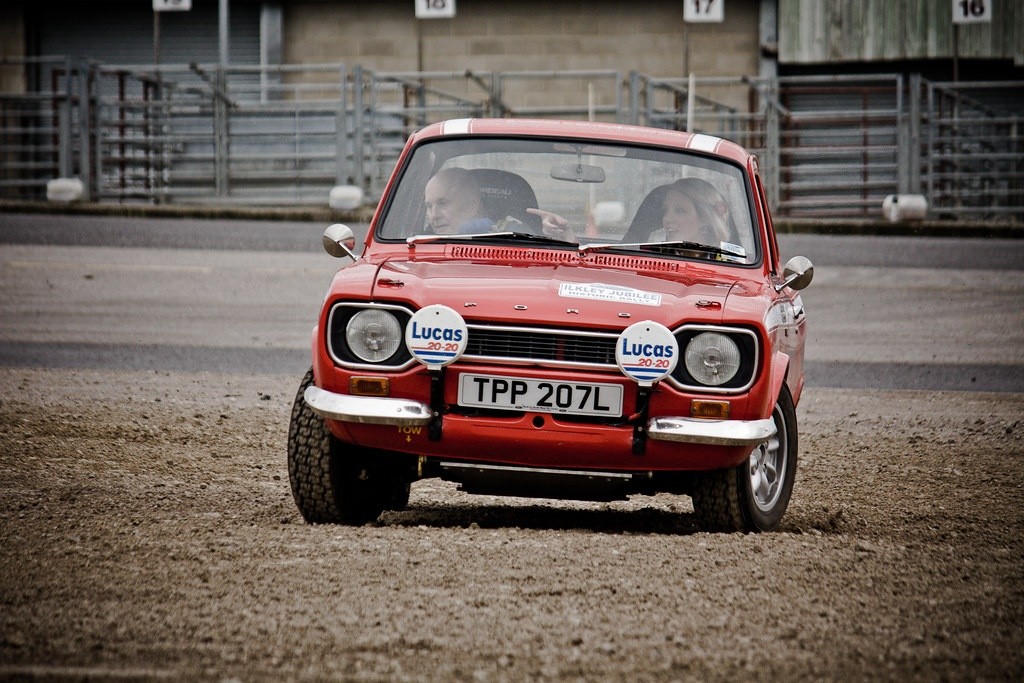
422;166;534;239
526;177;731;248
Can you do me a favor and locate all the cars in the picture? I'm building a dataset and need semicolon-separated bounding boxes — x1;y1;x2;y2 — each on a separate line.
287;120;816;537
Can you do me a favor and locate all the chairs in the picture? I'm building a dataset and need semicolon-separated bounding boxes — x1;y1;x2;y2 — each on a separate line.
421;168;545;237
617;184;744;250
